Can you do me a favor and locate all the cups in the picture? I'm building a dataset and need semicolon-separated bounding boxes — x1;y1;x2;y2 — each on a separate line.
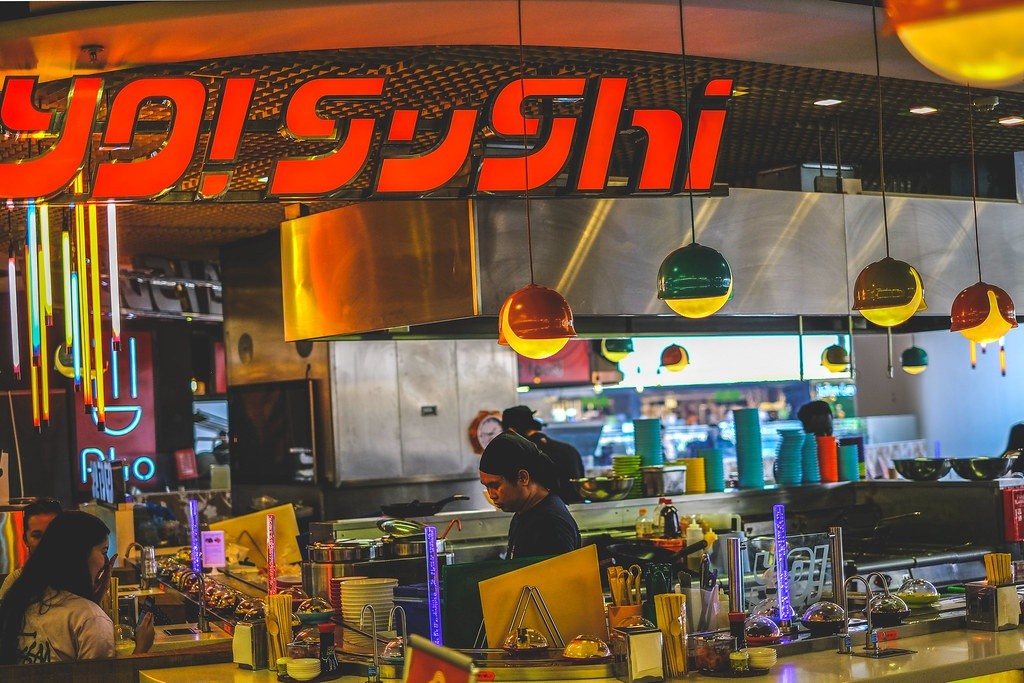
140;545;157;579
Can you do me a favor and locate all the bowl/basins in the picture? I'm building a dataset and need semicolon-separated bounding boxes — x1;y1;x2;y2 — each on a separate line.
950;456;1018;480
570;477;636;500
890;458;954;481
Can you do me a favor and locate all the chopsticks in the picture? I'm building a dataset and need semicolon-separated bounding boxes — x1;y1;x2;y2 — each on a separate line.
654;592;690;679
984;553;1013;587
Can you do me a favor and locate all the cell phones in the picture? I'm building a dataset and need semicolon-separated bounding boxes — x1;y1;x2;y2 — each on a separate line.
134;595;155;635
165;625;195;636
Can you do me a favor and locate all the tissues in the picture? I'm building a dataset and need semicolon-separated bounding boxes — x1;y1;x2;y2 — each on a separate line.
610;625;664;683
232;620;268;669
964;580;1022;632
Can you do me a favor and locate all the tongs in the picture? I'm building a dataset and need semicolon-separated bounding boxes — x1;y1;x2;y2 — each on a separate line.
606;565;643;607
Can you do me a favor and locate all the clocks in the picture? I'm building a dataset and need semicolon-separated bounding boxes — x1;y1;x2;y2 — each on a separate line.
467;409;507;452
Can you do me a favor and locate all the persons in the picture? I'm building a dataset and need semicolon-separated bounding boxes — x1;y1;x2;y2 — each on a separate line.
213;430;229;452
501;404;585;506
701;424;735;458
0;498;64;604
659;424;668;463
797;400;834;438
0;510;156;665
480;428;582;558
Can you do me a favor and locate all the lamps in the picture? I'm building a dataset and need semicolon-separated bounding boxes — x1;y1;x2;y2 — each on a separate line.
880;0;1023;88
951;78;1017;346
604;340;927;382
655;1;730;318
849;1;927;330
495;0;578;362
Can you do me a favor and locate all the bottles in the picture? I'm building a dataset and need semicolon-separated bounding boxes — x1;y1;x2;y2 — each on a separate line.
686;518;705;573
703;528;719;554
653;497;666;536
658;499;682;539
634;507;653;542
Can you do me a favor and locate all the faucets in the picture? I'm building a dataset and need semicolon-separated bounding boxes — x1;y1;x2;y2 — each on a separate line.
863;572;890;650
359;603;382;683
177;570;211;632
387;605;407;661
124;542;150;591
839;575;874;656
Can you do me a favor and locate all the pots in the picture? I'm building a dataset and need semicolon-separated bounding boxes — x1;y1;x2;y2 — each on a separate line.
305;536;448;563
379;495;473;517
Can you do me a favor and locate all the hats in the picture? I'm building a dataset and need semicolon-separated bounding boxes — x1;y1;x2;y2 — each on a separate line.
502;404;538;426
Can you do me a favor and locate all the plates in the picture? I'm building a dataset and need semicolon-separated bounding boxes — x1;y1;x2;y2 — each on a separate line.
329;576;399;632
611;407;868;498
286;658;321;682
740;647;779;671
